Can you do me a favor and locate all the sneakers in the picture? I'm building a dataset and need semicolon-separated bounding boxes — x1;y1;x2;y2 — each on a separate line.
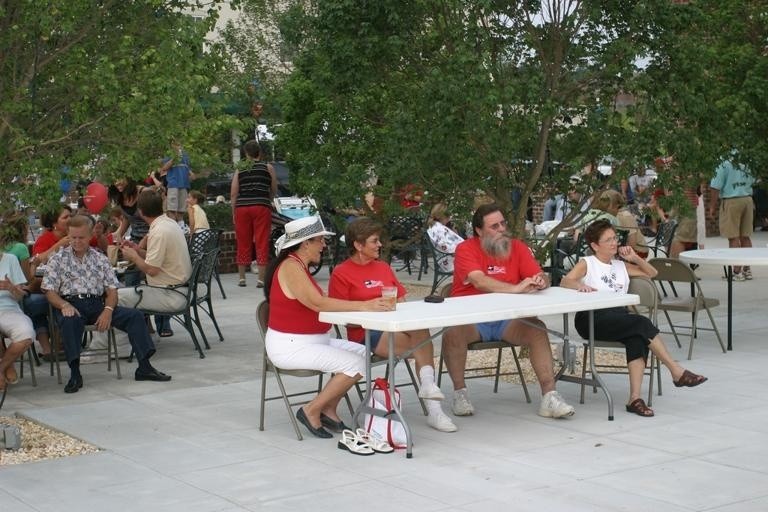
539;391;575;418
296;381;476;455
721;269;753;282
80;344;131;364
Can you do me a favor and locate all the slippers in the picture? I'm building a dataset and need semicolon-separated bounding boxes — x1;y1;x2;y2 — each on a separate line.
673;369;706;387
626;398;653;416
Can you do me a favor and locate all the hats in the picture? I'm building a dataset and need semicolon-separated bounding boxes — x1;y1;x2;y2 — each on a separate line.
275;217;336;256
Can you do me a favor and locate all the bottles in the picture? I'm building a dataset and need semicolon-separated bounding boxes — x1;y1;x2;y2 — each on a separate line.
177;149;183;162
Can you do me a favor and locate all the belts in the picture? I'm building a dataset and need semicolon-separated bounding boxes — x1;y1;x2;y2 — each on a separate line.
65;293;100;300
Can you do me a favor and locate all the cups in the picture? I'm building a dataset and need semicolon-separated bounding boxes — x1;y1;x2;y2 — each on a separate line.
107;246;118;267
382;285;397;312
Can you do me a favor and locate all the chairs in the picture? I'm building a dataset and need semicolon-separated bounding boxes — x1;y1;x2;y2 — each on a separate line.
438;281;532;403
372;216;468;281
645;257;727;359
330;266;429;417
0;228;227;386
255;300;361;441
579;276;662;407
425;221;678;298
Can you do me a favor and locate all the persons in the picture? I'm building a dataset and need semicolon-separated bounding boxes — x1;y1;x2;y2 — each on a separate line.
193;141;289;288
329;218;457;432
560;221;708;417
79;189;193;363
336;150;754;281
1;142;211;360
1;253;36;384
41;215;172;393
442;204;576;416
263;216;394;439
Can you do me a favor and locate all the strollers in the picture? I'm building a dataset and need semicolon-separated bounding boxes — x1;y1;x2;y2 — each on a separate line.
267;209;328;279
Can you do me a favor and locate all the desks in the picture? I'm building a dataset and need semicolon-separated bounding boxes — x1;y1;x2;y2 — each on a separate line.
679;247;768;350
318;287;640;457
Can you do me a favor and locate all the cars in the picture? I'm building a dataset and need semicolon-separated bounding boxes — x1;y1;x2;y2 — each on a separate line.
371;157;594;214
597;156;616;180
202;159;290;198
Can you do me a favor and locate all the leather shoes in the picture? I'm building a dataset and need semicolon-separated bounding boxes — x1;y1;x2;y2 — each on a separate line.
64;375;82;393
0;365;19;391
135;365;170;381
